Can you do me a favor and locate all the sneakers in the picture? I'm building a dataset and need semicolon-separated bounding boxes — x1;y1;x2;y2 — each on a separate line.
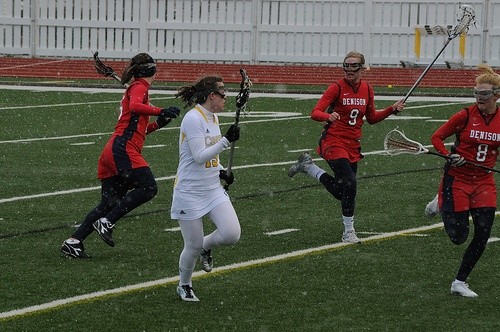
92;219;117;247
424;193;443;219
451;281;478;298
286;152;313;178
200;248;213;272
177;285;199;301
341;231;362;245
59;239;92;260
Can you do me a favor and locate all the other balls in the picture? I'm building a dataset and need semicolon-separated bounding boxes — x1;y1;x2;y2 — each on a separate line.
387;84;393;89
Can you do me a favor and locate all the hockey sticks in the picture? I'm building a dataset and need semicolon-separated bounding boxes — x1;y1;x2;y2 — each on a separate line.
393;4;476;116
224;68;253;191
94;50;171;122
384;129;500;174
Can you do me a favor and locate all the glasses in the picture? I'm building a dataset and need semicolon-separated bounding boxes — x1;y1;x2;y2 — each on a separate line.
213;87;226;97
473;86;500;99
343;63;363;73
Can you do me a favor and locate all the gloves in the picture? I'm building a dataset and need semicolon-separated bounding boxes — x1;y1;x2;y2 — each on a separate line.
161;106;181;118
224;124;239;142
448;154;466;168
219;168;233;184
157;115;173;127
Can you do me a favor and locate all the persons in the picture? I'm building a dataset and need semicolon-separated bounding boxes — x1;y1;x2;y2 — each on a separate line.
61;52;182;259
169;74;241;301
424;64;500;296
287;53;406;242
